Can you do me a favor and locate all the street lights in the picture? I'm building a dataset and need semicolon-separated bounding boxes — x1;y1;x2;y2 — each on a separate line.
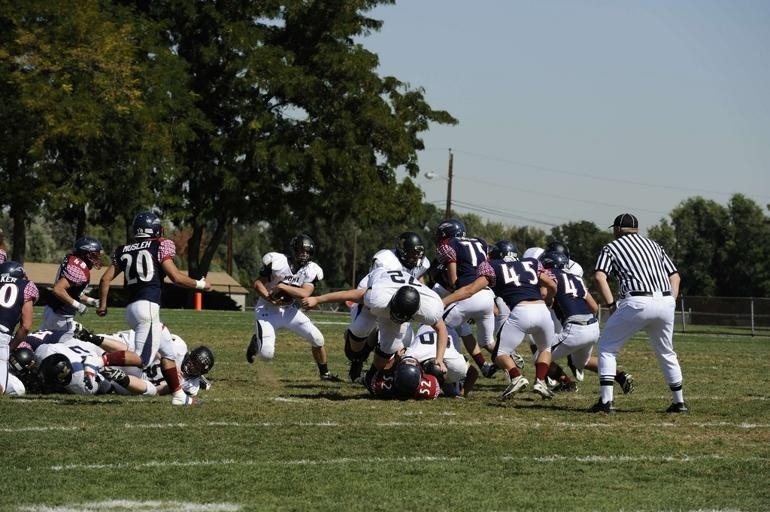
424;148;454;220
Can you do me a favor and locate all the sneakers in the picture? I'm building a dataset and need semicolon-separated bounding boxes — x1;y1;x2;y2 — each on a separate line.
482;351;585;397
81;354;98;392
171;398;197;408
666;402;687;413
622;373;633;393
350;362;363;381
246;334;257;365
586;396;616;414
320;373;344;382
73;324;88;340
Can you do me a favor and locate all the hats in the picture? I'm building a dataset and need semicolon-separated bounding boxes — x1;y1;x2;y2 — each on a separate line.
608;213;638;228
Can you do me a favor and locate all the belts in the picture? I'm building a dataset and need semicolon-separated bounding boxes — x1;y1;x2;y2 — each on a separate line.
629;291;671;296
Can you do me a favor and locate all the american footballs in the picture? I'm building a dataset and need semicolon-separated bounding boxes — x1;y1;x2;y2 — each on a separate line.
274;289;293;302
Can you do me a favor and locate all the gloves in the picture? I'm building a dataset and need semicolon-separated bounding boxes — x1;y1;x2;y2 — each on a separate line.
72;299;87;315
87;298;99;308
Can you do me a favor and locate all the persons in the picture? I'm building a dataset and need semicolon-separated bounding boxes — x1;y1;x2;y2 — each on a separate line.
245;211;686;413
1;210;216;405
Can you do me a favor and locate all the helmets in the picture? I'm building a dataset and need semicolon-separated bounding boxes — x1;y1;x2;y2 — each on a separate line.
41;353;72;386
131;212;162;238
0;261;26;279
74;237;104;270
388;286;420;324
181;345;214;379
396;231;425;269
487;241;570;269
290;234;315;263
397;357;447;400
436;219;464;243
9;348;34;376
